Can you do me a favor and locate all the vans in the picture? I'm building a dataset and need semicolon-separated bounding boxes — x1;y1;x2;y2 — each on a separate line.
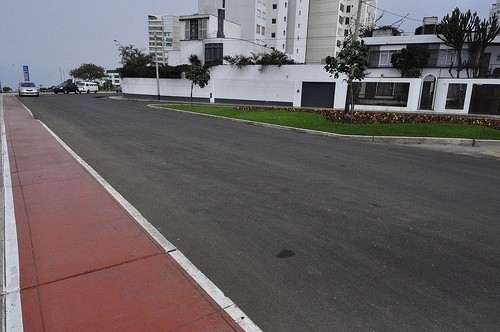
77;81;99;93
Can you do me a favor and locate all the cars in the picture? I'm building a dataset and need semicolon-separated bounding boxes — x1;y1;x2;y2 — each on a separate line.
53;82;78;94
18;81;39;97
